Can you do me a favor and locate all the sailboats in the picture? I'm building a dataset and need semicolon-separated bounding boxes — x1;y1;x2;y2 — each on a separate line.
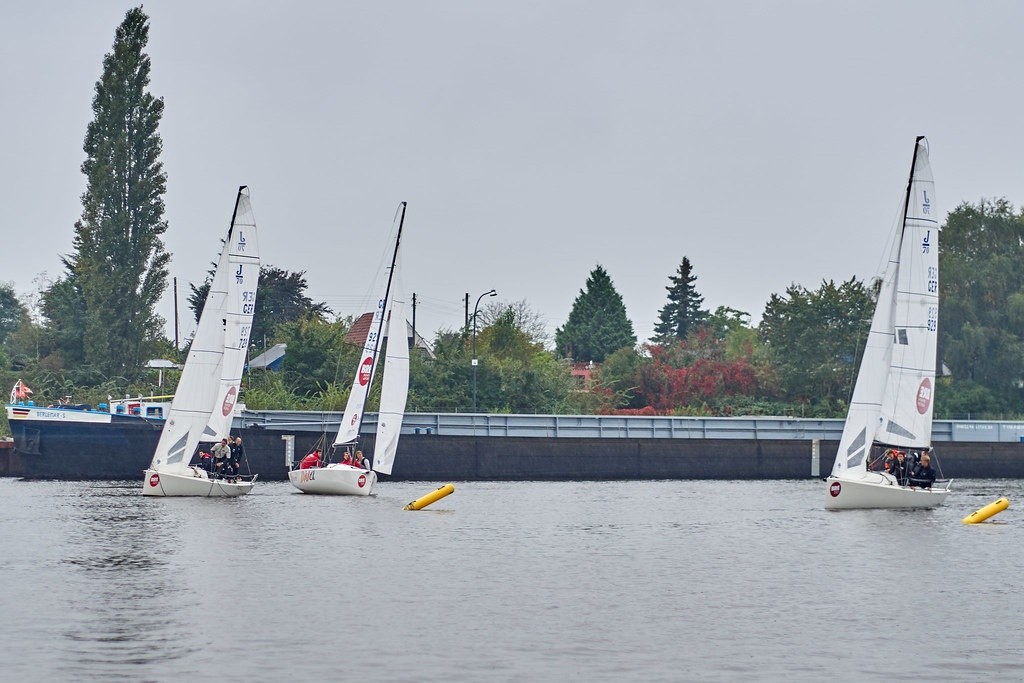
142;184;262;499
287;199;411;497
823;133;950;509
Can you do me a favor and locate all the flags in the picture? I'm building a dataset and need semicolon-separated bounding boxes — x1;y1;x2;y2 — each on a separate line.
20;381;33;394
15;386;30;400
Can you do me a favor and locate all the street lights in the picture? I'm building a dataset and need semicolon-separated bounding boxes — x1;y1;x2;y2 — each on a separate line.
473;289;497;412
247;344;256;390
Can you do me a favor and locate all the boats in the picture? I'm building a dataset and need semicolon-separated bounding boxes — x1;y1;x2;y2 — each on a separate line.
6;358;248;481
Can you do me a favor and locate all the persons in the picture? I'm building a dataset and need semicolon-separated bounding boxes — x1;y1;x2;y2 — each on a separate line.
198;448;215;478
341;452;362;468
356;450;370;470
300;449;323;469
47;401;54;408
884;449;936;489
211;435;244;480
